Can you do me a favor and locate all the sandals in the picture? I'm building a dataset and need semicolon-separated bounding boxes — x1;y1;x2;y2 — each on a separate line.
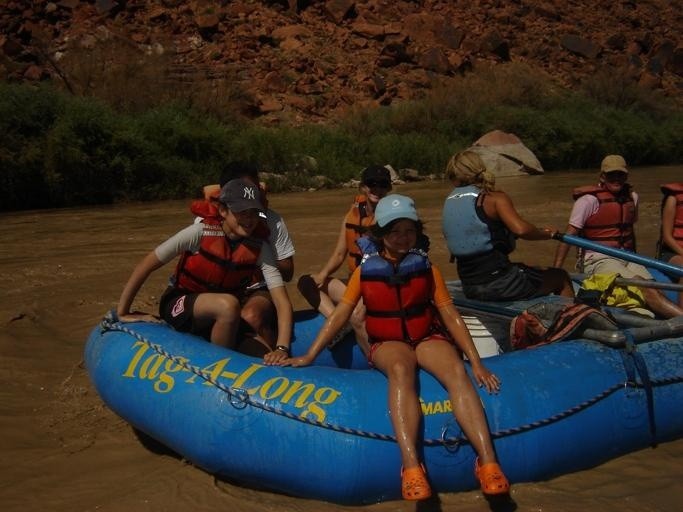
473;456;511;496
399;461;432;502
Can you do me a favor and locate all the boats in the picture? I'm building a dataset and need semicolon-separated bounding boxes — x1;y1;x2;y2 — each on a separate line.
83;267;681;507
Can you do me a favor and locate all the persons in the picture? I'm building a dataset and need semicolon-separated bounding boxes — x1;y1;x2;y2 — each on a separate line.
113;176;294;367
555;155;682;321
271;193;509;501
439;149;576;304
192;160;297;349
655;174;682;307
295;166;392;350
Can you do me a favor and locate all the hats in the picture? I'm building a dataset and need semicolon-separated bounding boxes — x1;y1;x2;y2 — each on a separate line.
218;179;267;216
600;154;629;175
361;165;392;188
375;194;418;229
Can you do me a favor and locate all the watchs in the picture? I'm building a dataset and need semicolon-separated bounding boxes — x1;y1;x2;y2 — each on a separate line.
274;345;288;354
